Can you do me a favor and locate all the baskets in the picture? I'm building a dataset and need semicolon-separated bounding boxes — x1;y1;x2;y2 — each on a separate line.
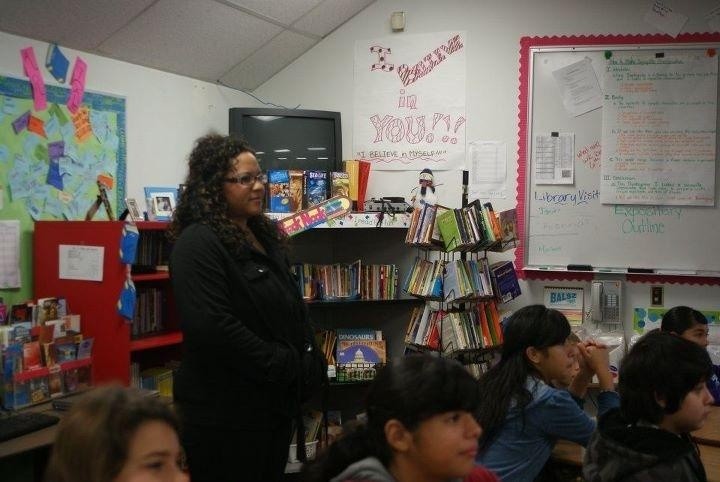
335;362;389;382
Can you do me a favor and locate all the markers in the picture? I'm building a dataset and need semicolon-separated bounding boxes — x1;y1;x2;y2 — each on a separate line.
539;267;548;269
599;270;612;272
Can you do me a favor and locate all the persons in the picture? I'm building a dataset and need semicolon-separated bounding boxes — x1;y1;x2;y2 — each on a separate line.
298;354;499;482
582;328;715;481
469;304;621;481
158;198;172;211
170;133;345;482
660;306;719;407
44;380;192;482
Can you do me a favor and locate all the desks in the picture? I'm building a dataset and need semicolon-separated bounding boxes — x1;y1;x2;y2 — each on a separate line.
552;383;720;482
0;389;178;460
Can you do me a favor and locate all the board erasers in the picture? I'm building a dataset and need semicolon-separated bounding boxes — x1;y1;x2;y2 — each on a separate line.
567;264;593;271
628;268;653;273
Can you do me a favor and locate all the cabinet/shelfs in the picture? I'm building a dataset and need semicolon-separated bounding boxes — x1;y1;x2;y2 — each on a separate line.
33;221;184;388
263;213;428;474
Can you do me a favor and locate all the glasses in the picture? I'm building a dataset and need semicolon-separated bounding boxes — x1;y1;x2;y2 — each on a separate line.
222;174;269;186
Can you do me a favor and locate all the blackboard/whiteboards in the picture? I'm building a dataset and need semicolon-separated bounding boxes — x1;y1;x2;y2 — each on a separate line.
522;42;720;278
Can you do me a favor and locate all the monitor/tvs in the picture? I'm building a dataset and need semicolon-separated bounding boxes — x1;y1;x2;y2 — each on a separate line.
229;107;342;202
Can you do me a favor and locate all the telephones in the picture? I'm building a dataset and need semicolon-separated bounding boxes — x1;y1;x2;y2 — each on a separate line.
590;279;624;325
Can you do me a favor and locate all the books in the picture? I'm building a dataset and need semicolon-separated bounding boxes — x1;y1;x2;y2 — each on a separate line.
403;200;522;355
130;231;170;272
464;360;497;381
0;286;183;412
290;402;369;452
269;159;371;211
295;260;398;300
317;329;386;381
